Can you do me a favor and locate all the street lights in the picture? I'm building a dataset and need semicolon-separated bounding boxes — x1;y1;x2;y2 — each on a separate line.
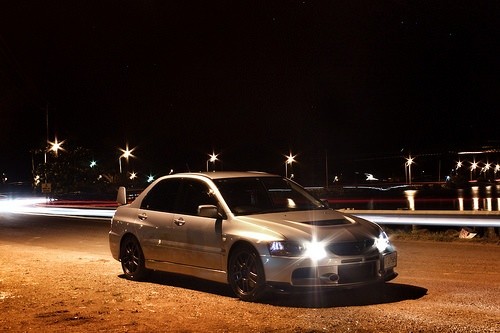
285;157;294;178
404;158;413;182
469;162;477;180
45;142;60;203
206;151;219;172
119;151;130;173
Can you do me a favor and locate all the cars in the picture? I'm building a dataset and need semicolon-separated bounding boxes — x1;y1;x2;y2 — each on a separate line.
108;170;398;301
